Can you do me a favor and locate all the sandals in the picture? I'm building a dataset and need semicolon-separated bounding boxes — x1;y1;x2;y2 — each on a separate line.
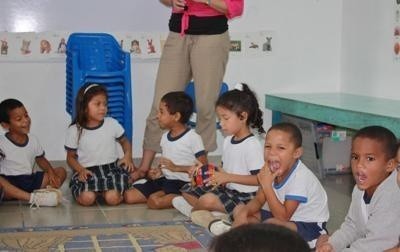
128;168;148;184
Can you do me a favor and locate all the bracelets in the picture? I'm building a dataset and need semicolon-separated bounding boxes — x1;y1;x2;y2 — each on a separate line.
207;0;210;7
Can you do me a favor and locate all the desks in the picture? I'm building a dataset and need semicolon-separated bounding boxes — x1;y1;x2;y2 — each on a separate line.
264;91;400;180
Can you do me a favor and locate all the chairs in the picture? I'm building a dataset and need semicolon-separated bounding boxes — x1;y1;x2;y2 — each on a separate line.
65;33;132;144
182;81;228;129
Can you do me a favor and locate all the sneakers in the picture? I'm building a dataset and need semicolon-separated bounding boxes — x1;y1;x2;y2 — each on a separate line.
132;179;147;186
191;210;227;227
171;197;192;216
208;219;231;236
30;188;62;208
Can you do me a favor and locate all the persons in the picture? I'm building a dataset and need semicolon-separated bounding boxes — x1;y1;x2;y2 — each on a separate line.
0;99;67;206
315;123;400;252
133;0;246;173
382;143;400;252
171;82;264;224
62;82;134;205
122;90;209;210
192;119;331;249
